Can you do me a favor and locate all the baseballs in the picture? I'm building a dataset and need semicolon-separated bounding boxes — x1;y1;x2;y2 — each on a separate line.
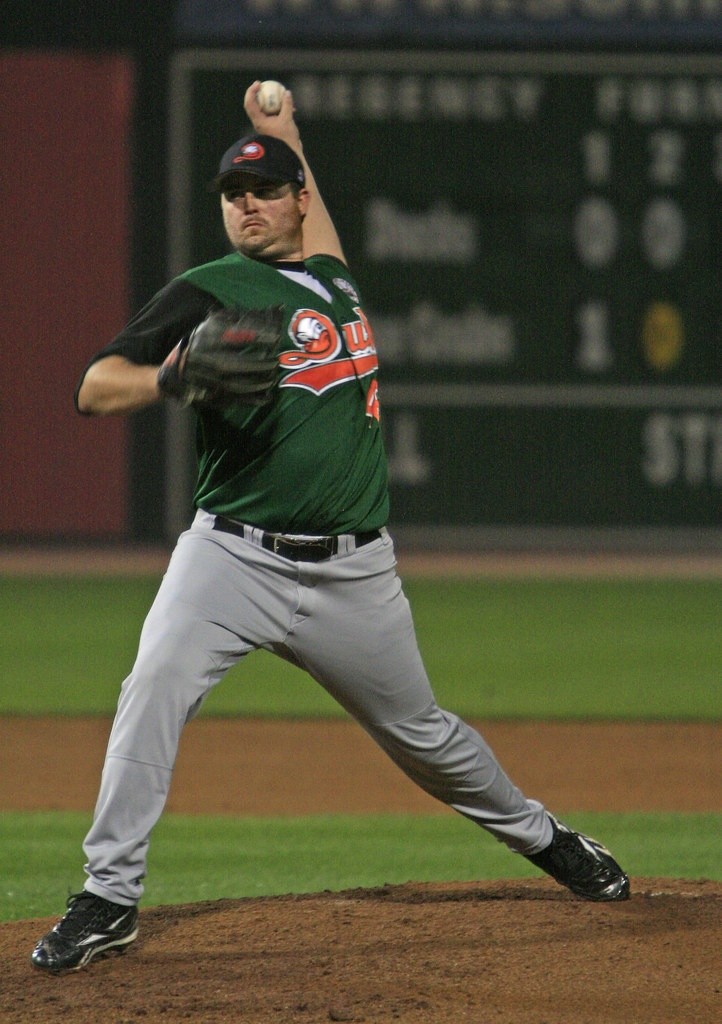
252;78;290;117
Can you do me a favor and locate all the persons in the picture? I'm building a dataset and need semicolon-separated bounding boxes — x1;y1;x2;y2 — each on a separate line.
30;79;631;975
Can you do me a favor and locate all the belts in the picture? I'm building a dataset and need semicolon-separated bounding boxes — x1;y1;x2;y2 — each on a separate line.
211;512;381;565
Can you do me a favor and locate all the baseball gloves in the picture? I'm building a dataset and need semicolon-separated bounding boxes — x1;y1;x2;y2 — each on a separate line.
153;300;294;415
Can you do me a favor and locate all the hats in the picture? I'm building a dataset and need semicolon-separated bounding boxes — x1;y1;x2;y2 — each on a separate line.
208;132;307;198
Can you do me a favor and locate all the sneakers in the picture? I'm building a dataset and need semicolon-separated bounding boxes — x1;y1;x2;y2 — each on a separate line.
514;809;633;904
28;884;142;975
